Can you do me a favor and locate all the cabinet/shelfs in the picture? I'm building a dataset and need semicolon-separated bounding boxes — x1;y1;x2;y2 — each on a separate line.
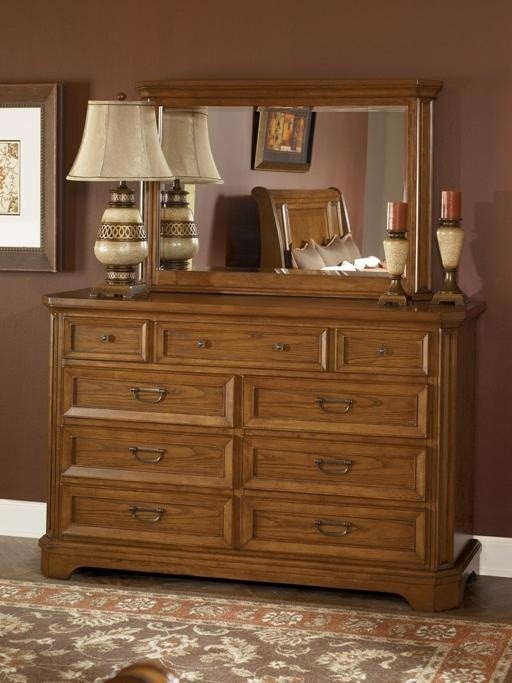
36;286;488;616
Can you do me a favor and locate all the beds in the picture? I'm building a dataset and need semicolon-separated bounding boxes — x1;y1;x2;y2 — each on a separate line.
252;185;388;274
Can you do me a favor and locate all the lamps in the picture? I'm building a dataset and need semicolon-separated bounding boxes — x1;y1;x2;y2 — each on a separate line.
65;92;176;300
159;104;225;271
376;201;415;230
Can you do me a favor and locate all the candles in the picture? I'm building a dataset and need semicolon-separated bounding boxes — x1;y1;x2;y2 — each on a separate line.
430;188;473;217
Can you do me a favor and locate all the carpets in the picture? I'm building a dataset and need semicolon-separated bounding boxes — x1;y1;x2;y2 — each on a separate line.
1;579;512;682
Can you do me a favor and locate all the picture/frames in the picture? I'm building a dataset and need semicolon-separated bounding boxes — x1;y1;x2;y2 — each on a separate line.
252;104;321;173
1;82;67;274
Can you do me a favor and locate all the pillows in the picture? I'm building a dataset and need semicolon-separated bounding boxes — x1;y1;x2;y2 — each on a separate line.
289;231;363;273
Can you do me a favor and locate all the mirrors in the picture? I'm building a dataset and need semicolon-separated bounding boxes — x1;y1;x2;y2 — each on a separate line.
136;76;442;299
